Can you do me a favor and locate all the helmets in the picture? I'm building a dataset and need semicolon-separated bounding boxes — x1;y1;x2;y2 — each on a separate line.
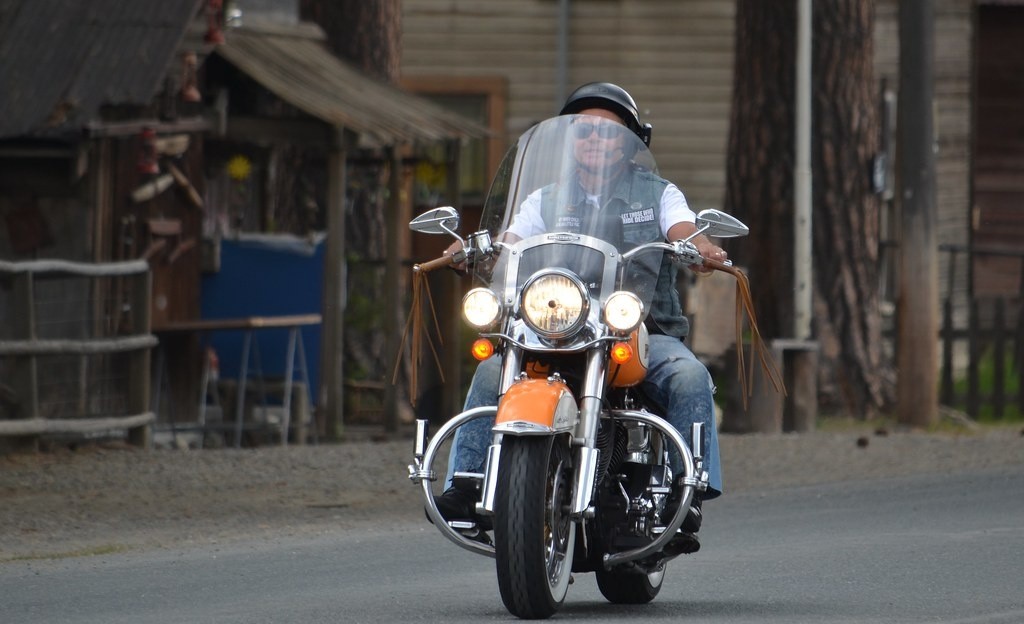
558;82;653;151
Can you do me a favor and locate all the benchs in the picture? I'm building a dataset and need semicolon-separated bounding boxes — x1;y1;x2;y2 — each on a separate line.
722;339;822;436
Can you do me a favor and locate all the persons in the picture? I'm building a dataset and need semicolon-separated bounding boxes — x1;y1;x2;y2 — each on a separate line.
425;83;729;532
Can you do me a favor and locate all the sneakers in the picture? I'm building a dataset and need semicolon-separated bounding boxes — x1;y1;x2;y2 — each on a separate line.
659;478;704;533
424;477;494;532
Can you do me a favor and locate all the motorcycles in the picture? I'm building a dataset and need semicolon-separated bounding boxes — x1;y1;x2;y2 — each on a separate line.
403;114;753;617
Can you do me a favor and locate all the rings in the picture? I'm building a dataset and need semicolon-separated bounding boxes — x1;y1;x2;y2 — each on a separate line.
443;251;447;253
715;251;727;256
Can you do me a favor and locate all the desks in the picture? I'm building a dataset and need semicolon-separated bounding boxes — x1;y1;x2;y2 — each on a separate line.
149;314;324;450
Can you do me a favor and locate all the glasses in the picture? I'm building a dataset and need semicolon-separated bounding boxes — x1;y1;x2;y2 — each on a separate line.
571;122;628;140
